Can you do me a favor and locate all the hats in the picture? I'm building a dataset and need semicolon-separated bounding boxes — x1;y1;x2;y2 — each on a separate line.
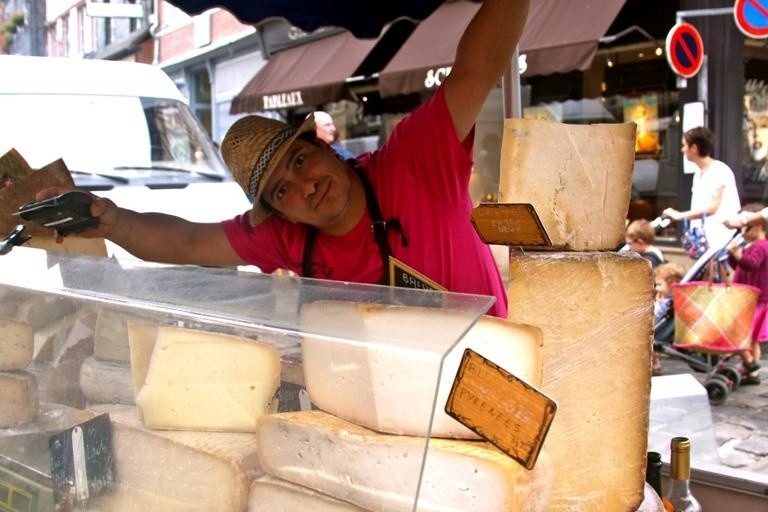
221;112;317;228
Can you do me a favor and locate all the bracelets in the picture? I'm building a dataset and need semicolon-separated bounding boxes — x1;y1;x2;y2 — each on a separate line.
743;217;750;228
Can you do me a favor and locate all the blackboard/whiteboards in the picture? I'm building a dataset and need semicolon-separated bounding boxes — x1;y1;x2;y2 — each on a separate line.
50;413;112;510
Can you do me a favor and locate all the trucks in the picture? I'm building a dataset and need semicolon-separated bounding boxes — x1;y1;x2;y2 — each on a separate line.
0;52;266;282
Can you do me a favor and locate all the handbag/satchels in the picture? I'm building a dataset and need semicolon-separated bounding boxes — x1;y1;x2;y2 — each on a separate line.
670;257;761;354
682;211;709;259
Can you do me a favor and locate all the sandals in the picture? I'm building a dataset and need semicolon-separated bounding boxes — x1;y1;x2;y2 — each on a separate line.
742;359;760;386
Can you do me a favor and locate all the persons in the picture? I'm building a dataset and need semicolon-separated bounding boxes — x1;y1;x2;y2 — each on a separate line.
621;216;665;268
33;0;532;323
660;125;742;281
726;202;768;386
720;204;768;232
649;260;688;377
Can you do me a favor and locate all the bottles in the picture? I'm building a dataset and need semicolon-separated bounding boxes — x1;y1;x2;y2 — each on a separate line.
664;436;702;512
645;452;675;512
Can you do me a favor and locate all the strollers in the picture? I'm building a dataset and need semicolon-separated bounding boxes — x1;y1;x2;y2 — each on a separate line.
615;208;767;404
